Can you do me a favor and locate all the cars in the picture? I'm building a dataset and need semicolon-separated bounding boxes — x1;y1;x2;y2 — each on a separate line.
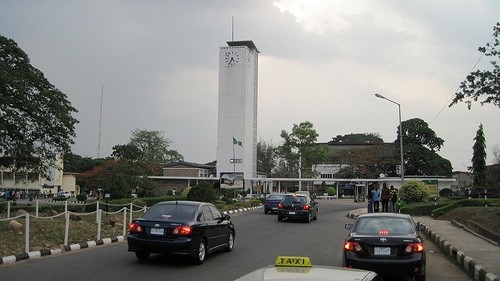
342;213;426;281
278;194;318;223
58;191;71;199
295;191;314;201
264;193;285;214
234;255;383;280
127;201;236;264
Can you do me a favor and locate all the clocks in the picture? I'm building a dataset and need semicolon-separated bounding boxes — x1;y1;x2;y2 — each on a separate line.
224;51;240;66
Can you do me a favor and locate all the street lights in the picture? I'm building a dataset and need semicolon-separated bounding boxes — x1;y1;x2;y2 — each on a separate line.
374;92;404;186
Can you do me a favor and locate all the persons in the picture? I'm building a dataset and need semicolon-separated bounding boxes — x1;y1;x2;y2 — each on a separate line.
371;186;380;213
0;188;27;199
389;184;398;213
380;182;391;213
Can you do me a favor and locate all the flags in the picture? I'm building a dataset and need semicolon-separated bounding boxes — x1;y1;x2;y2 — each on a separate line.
233;137;242;147
